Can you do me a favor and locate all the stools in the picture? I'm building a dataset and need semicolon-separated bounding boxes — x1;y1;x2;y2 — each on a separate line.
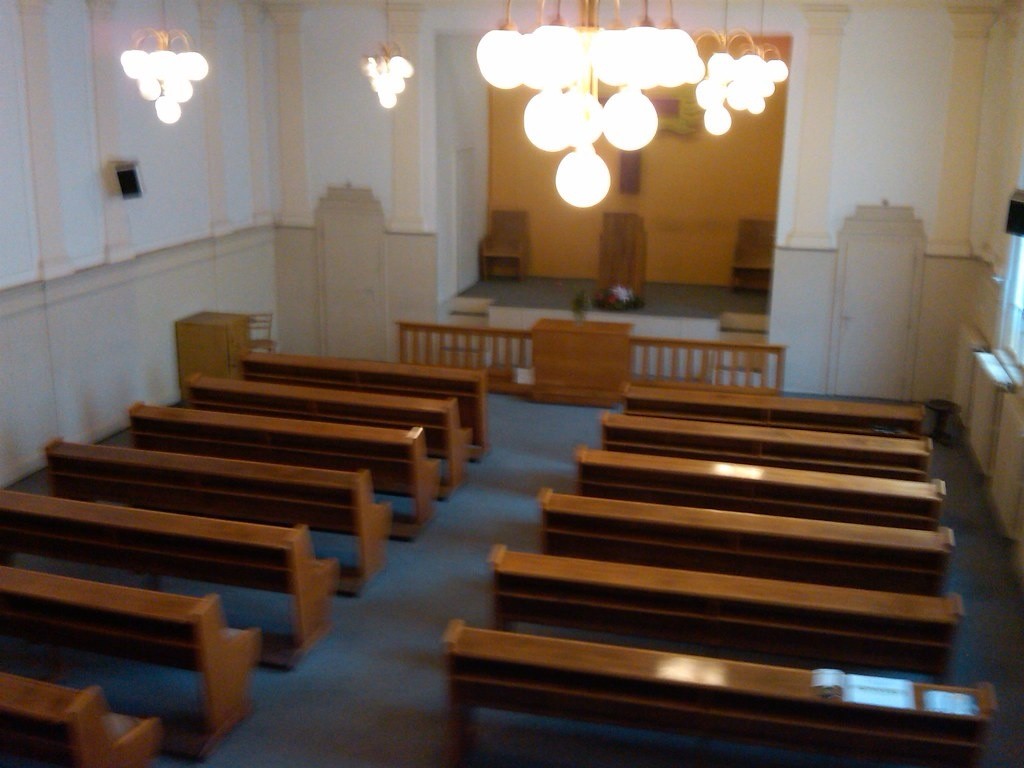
926;399;962;449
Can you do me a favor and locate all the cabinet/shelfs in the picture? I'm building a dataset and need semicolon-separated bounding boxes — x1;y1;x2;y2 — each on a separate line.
175;311;251;402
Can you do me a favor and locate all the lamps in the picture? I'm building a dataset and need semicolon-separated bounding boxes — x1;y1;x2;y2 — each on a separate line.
362;0;414;111
688;0;788;136
120;1;209;126
475;0;706;208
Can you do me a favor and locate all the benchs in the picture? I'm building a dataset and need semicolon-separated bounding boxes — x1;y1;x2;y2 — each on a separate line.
0;349;998;768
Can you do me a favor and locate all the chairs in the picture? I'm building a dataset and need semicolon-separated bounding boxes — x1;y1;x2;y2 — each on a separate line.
251;313;278;353
727;217;776;293
479;210;530;284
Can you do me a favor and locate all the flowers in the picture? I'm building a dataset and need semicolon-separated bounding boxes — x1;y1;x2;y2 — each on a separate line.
589;283;648;312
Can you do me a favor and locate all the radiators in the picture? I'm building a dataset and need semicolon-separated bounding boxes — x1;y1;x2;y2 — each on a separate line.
950;320;1015;478
